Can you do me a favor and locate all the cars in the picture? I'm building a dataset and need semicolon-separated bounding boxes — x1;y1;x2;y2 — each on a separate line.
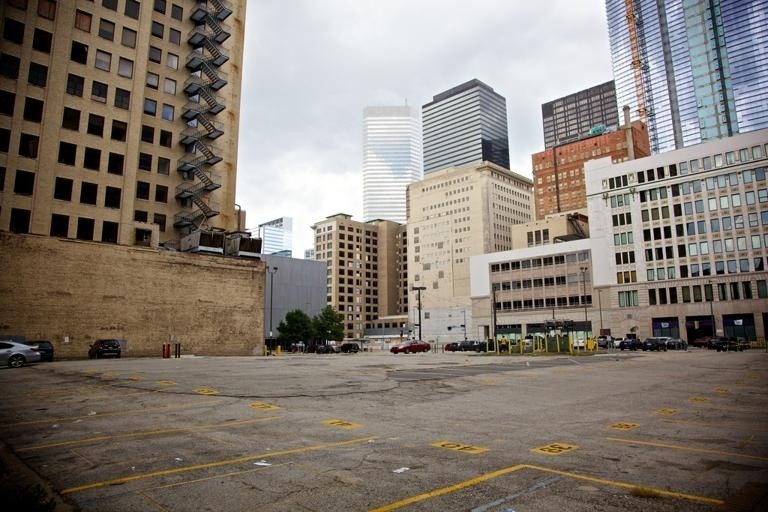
288;343;359;353
390;341;430;354
445;335;544;353
573;335;751;352
88;339;121;358
0;340;54;368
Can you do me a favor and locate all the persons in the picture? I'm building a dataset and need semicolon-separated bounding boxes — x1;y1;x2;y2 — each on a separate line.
500;336;507;352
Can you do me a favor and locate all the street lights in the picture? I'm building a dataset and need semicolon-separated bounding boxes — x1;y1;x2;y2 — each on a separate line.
579;266;588;341
412;286;426;338
265;265;277;338
